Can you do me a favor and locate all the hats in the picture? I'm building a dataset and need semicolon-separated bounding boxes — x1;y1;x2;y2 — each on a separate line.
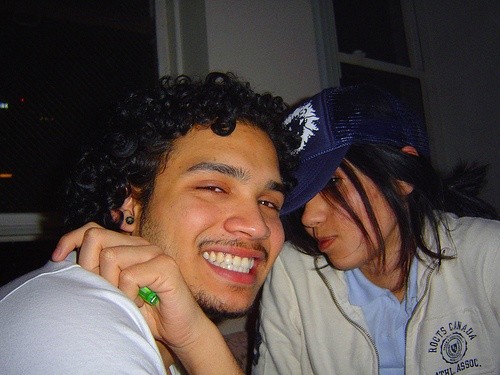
279;83;433;218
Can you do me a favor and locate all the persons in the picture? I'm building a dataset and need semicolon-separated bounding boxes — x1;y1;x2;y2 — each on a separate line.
0;70;298;375
235;86;499;375
